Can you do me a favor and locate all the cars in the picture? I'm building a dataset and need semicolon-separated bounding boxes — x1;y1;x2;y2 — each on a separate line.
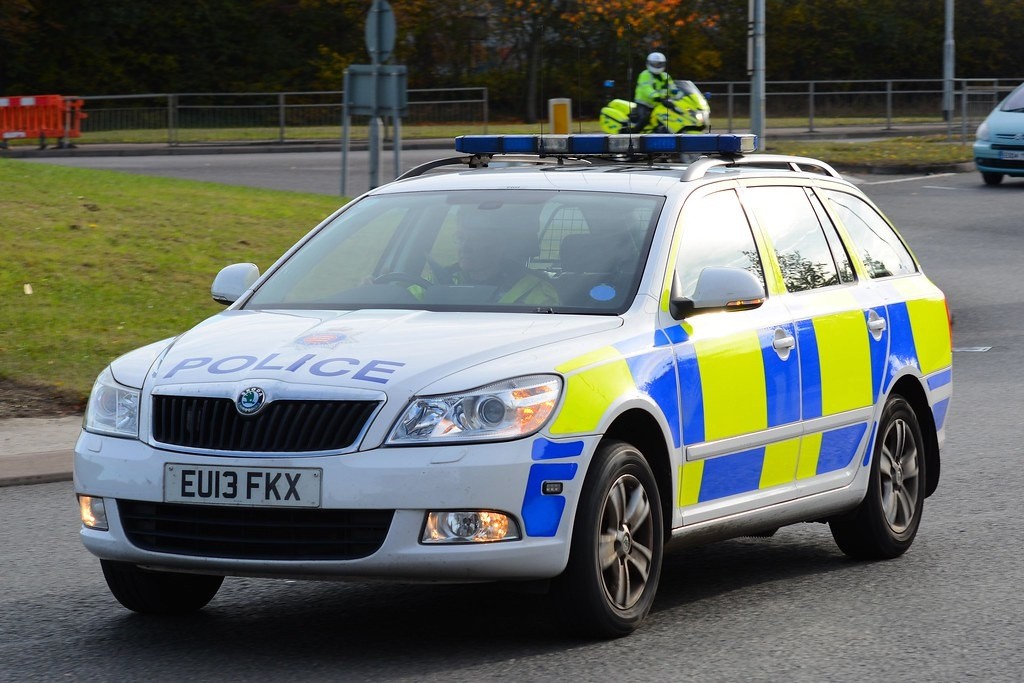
972;83;1024;186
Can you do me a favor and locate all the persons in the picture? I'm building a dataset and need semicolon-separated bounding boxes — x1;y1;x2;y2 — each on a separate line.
630;52;678;134
406;221;561;307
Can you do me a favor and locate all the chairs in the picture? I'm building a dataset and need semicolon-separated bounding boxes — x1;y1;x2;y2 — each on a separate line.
557;230;636;309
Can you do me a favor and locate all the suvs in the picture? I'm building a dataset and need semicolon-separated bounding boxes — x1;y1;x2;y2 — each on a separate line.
72;133;953;644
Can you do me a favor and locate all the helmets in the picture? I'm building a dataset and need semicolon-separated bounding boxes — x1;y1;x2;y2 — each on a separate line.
646;52;666;75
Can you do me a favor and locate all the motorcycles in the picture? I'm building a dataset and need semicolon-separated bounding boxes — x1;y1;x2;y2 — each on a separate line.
598;80;712;134
